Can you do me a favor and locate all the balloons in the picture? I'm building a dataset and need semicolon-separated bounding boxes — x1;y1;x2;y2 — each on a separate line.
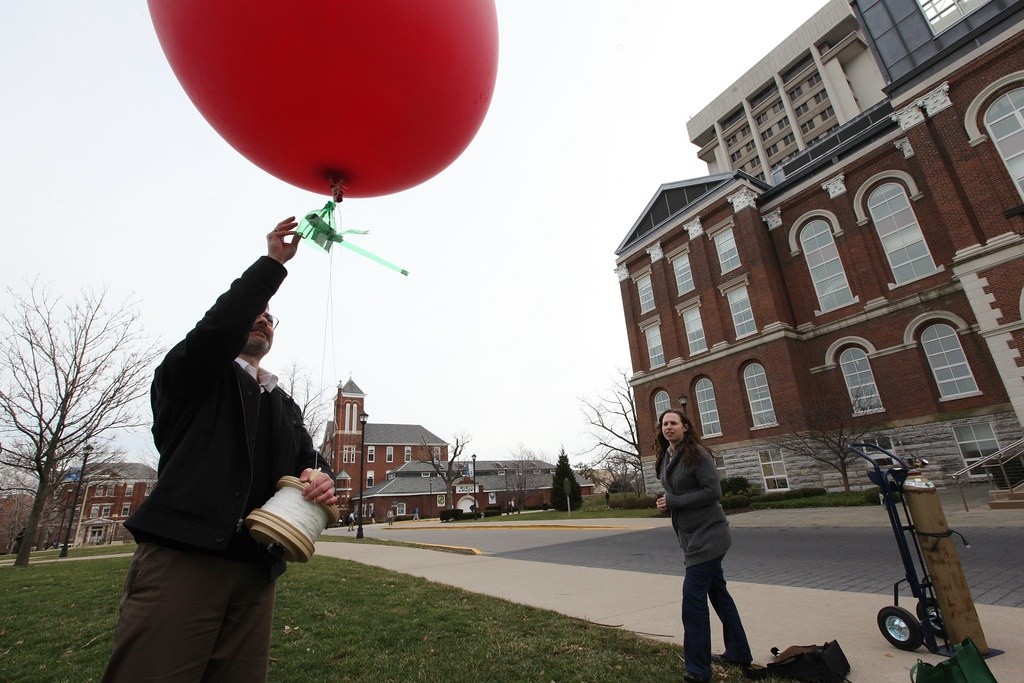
149;0;500;205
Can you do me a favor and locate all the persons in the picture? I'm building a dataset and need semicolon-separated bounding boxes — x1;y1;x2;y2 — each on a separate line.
371;511;376;524
387;509;394;525
99;217;339;683
16;528;25;552
605;491;609;506
653;409;753;683
340;509;356;531
412;506;420;521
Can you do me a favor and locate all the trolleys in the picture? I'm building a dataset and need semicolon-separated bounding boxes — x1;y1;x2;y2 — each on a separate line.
848;442;1005;660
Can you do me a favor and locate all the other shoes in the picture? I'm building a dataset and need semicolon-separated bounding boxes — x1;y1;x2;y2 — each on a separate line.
684;669;711;682
712;651;751;666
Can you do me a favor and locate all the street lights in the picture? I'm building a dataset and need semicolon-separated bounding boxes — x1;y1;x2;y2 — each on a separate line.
58;444;94;557
504;465;510;515
356;409;369;538
471;454;478;519
55;485;73;549
678;393;688;417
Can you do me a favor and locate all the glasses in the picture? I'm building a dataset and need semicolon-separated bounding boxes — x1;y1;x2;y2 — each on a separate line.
262;313;272;322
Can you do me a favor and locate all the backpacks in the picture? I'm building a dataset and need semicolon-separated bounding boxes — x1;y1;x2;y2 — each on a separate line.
768;640;850;683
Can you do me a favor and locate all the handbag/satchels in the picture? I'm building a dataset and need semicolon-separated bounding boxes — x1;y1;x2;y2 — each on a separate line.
916;637;997;683
